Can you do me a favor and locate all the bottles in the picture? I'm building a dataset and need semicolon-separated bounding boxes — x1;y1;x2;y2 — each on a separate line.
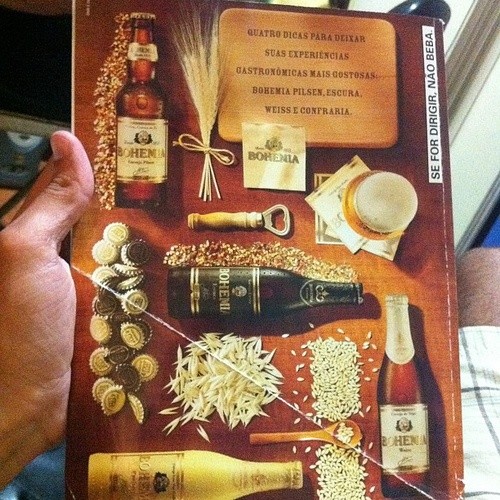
114;12;170;208
376;294;432;499
86;449;305;500
166;267;365;319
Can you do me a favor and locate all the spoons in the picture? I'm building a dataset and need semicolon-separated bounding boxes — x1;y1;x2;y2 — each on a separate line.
250;420;362;448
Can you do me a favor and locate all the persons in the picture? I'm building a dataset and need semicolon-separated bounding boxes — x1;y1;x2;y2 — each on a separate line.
0;131;500;500
